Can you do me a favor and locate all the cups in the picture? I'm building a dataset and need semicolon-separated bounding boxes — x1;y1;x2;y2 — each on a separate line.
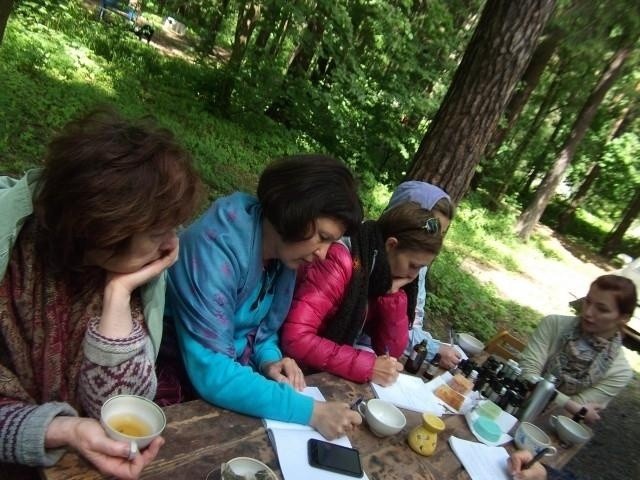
221;458;277;480
99;395;168;462
358;398;407;438
549;414;590;449
514;422;557;459
455;332;485;355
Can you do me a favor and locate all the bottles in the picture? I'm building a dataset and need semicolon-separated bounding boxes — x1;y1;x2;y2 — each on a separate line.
451;357;558;421
401;339;428;375
426;355;443;379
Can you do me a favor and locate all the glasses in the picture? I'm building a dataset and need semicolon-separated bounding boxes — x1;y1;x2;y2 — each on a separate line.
383;217;443;235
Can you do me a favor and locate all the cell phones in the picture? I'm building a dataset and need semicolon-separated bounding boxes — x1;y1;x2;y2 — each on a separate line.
308;438;364;478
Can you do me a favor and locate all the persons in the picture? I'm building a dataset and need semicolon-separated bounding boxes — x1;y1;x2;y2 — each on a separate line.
507;451;577;479
519;274;637;426
360;180;461;371
161;154;364;440
280;201;443;386
0;110;202;480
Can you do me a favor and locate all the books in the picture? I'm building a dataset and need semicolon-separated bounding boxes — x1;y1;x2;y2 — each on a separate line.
443;342;469;363
263;385;367;480
370;372;441;416
446;437;512;479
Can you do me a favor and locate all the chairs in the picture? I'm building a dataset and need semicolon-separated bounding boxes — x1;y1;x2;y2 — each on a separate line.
133;25;154;47
483;332;528;365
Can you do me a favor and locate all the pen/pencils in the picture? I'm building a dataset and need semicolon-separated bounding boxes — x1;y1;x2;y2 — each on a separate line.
450;327;454;346
509;447;550;480
351;396;364;410
384;345;390;360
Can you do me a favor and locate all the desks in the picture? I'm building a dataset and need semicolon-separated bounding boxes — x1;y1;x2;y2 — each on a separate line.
38;354;596;480
97;5;136;31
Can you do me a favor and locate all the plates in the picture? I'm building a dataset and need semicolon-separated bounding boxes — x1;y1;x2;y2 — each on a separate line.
206;466;221;480
